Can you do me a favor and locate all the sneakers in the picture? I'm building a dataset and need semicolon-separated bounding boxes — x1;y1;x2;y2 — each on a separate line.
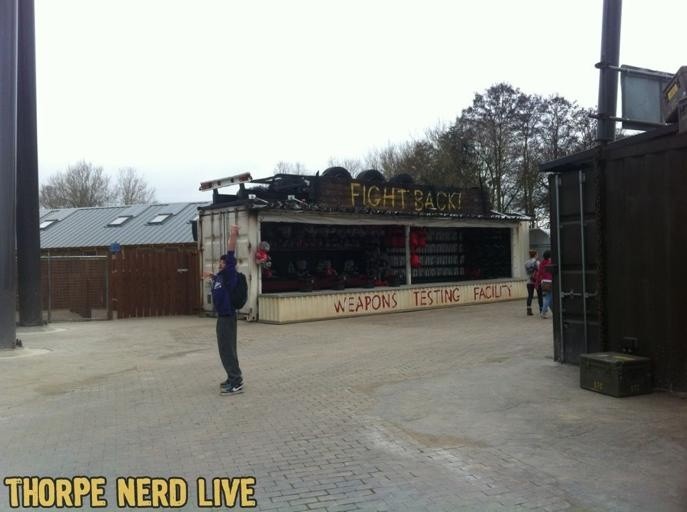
221;378;247;395
541;312;549;319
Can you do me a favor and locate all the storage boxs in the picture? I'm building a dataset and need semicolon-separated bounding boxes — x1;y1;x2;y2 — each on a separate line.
580;350;652;398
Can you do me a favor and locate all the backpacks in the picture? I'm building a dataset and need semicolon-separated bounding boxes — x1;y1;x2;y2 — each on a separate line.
231;273;249;309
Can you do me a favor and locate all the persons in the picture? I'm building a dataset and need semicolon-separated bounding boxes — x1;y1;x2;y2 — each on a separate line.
524;250;554;319
289;246;392;290
262;257;273;293
201;223;248;395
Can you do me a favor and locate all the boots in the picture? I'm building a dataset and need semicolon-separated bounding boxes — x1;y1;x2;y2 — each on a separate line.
528;308;533;315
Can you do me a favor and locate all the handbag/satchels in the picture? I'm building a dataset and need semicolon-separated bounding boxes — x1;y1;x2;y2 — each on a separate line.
529;259;538;283
